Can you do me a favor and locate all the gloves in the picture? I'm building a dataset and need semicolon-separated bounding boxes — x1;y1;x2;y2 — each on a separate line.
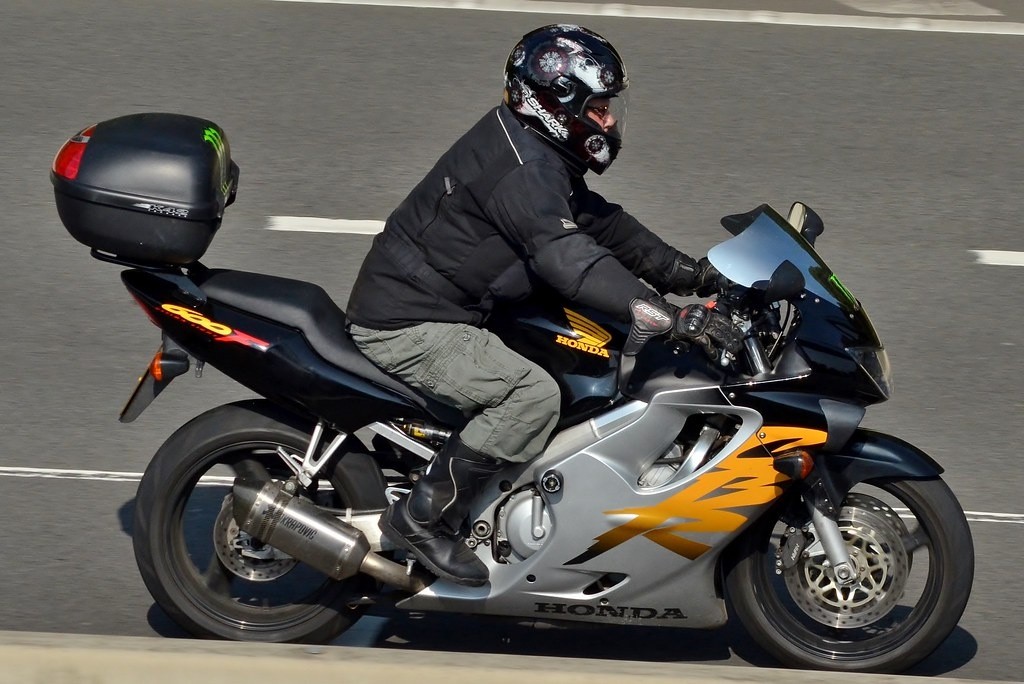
666;243;731;299
623;282;747;381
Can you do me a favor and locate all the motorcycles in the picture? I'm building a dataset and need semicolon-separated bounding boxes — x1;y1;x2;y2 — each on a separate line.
48;111;976;674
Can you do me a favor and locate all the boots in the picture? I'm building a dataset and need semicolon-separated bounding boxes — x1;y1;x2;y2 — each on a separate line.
379;430;529;587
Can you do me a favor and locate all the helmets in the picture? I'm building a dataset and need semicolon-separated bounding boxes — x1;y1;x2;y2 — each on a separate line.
503;24;630;176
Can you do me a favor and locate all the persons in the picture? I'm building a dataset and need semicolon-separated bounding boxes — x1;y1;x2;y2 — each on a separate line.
344;23;733;588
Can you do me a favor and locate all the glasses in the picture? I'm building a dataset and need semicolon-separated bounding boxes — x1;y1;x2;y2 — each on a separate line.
590;103;610;119
